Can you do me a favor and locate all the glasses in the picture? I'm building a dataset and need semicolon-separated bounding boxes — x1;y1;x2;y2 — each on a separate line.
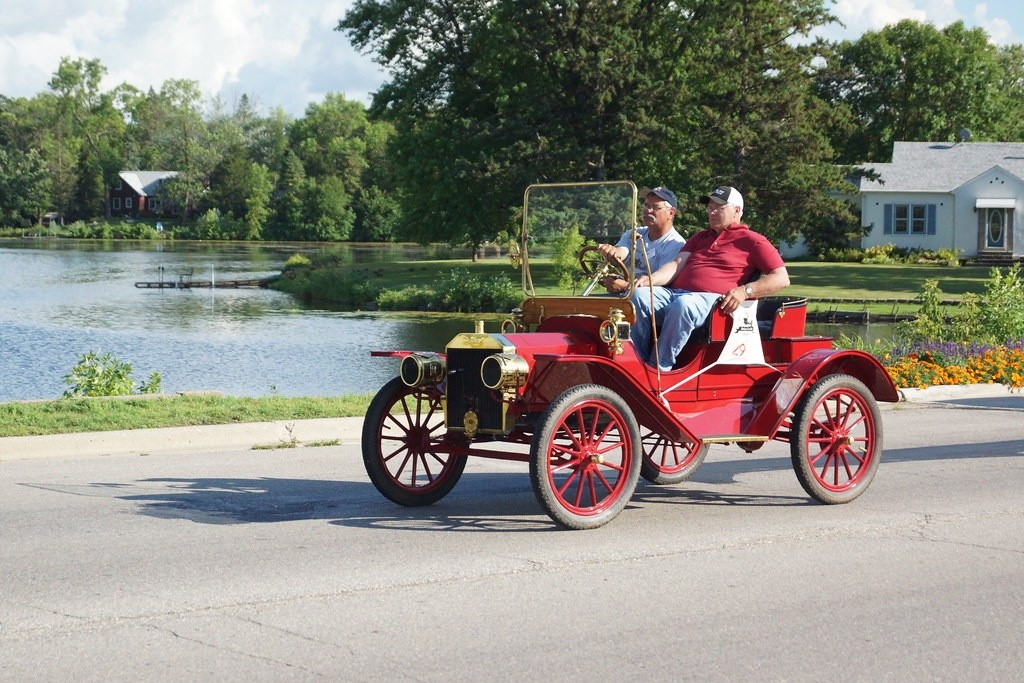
705;206;737;213
641;204;669;211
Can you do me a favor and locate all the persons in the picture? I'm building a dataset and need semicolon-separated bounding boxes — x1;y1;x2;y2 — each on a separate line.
623;185;791;372
596;187;687;297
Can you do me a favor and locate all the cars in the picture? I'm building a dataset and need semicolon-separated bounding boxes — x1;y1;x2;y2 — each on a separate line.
361;180;904;528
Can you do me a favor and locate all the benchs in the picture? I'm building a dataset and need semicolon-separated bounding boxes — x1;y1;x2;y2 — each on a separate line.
691;296;808;343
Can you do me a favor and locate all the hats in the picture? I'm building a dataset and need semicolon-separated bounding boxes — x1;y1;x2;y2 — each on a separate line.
639;187;678;209
700;186;744;211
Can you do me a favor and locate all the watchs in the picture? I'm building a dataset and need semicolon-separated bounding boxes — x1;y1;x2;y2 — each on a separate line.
744;283;755;299
